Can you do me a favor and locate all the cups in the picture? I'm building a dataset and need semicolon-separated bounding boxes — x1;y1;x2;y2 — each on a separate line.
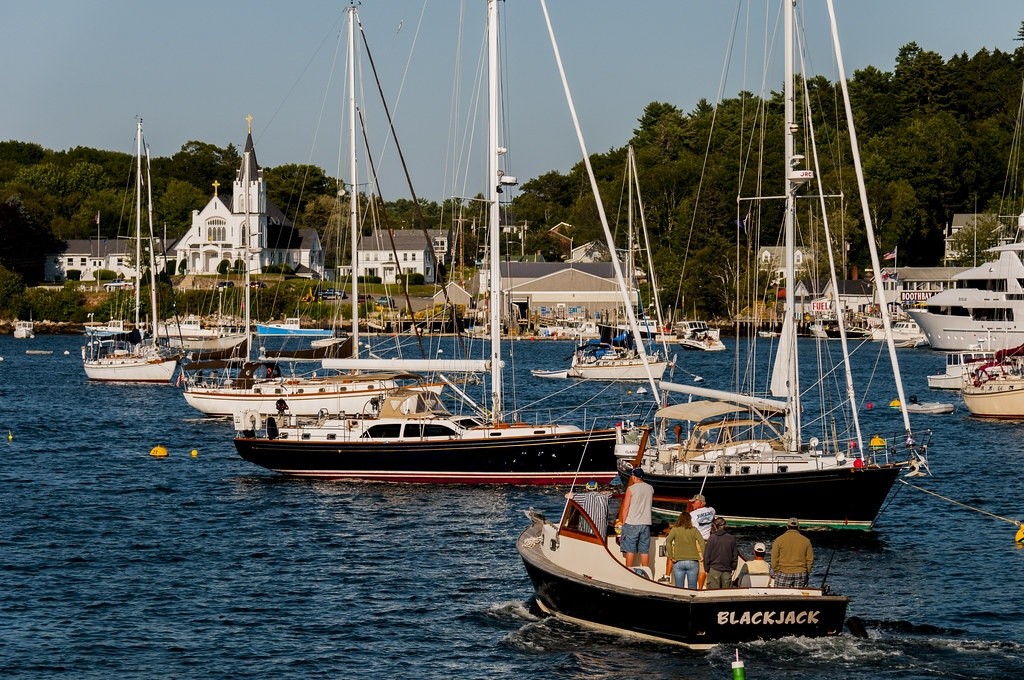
732;661;745;680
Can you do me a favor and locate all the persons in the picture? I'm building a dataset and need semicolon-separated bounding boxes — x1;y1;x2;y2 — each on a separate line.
735;540;777;586
665;511;706;590
194;369;203;387
688;494;716;589
702;519;740;589
209;368;219;385
769;518;814;589
660;520;678;584
619;468;654;567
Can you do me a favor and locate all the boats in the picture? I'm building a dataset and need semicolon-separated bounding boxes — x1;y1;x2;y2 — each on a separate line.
13;321;35;338
517;489;851;651
84;151;354;367
464;302;708;346
808;308;924;342
898;401;954;416
927;329;1005;391
679;327;726;352
757;330;778;340
960;343;1023;421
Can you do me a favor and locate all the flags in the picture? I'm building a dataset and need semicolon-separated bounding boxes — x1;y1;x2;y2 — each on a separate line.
92;211;99;224
883;248;896;260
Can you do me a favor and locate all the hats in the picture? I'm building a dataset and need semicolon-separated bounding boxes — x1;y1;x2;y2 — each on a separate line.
586;481;598;490
787;517;799;526
631;467;644;478
689;495;705;503
754;543;766;552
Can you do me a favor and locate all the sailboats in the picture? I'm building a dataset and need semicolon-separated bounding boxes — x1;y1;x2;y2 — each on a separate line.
178;1;451;423
80;116;180;385
528;144;677;380
612;0;928;535
230;1;656;490
905;101;1024;351
